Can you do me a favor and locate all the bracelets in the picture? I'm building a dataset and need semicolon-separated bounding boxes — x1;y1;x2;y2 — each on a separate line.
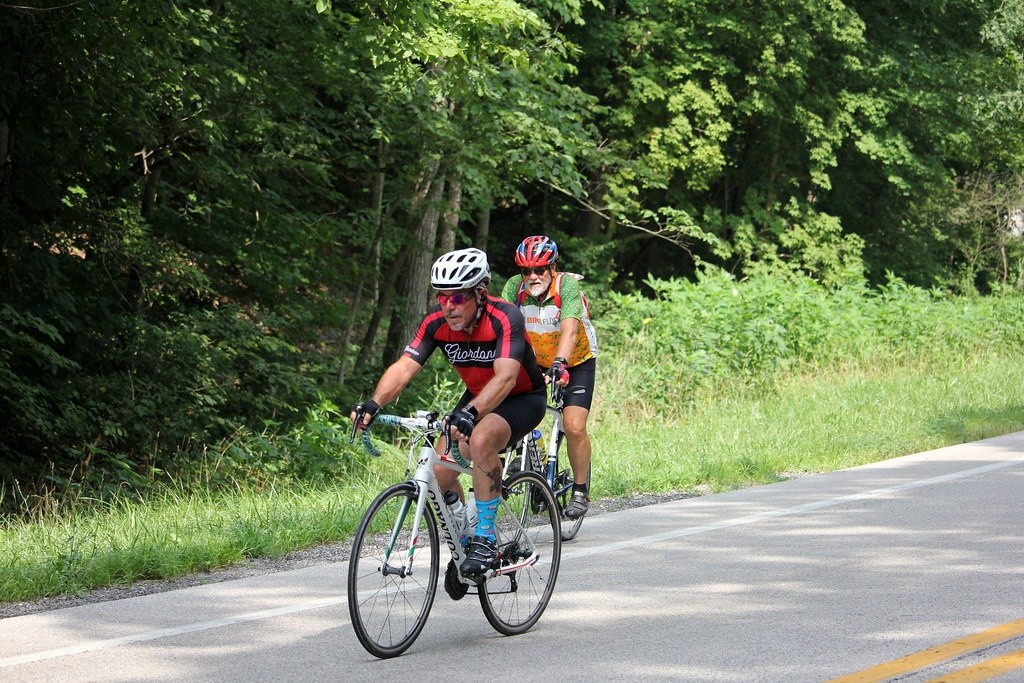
553;356;568;365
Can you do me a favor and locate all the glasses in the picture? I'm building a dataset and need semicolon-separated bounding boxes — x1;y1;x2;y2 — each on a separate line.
522;265;550;275
435;291;476;305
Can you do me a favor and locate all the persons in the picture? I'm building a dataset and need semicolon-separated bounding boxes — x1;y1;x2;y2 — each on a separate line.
499;236;600;518
350;248;548;575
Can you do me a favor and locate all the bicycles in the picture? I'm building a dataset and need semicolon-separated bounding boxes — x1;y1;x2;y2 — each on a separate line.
347;401;562;660
491;367;592;547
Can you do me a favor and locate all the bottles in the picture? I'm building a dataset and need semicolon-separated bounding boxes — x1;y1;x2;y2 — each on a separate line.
465;487;478;535
534;430;548;464
444;489;470;536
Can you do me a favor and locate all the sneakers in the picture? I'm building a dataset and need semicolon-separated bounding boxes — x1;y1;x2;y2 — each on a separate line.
507;457;522;473
460;536;498;577
566;491;589;518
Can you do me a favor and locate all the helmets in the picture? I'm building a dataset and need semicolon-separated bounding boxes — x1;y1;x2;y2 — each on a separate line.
514;235;558;268
430;248;491;290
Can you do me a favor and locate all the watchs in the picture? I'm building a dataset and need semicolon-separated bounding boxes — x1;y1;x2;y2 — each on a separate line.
464;404;479;421
